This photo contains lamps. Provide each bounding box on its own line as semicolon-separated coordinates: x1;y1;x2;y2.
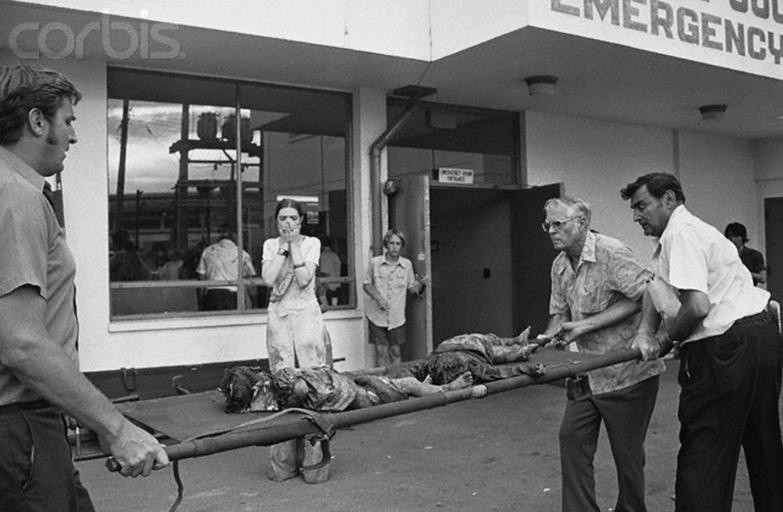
699;104;727;122
521;74;559;98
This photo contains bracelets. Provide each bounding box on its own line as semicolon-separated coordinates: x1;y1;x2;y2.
278;248;289;258
666;334;686;348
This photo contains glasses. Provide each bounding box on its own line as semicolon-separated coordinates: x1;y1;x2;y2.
542;215;581;233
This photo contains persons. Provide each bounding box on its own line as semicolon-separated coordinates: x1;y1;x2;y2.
0;62;170;511
262;201;334;482
319;236;342;304
361;229;428;369
198;232;255;310
222;325;535;417
724;221;765;286
108;225;152;283
537;197;663;509
621;172;780;511
157;249;183;279
